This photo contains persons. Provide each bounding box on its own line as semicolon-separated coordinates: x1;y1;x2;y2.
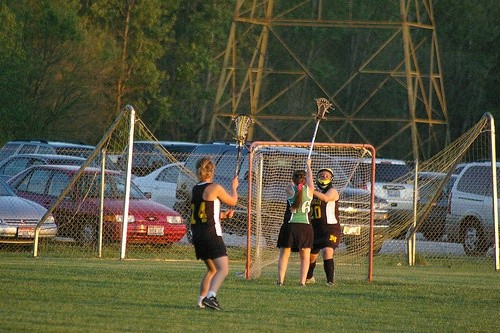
305;166;341;286
189;157;239;311
276;158;315;287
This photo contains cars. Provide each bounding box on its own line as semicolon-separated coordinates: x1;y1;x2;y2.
0;178;58;247
411;171;459;239
133;162;188;210
0;154;102;183
5;164;188;249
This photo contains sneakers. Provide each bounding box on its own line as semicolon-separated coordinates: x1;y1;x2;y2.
202;297;221;310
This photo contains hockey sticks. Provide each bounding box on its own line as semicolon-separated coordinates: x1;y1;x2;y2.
226;113;256;221
303;97;336;173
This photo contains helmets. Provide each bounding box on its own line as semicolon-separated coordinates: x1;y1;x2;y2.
316;168;334;188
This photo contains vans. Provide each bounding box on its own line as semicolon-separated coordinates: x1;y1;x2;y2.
443;162;500;256
114;139;211;176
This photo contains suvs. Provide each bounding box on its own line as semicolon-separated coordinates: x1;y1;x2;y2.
333;156;420;238
173;140;390;256
0;136;120;171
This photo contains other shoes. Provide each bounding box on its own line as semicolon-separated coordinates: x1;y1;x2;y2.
325;282;333;286
306;278;315;283
